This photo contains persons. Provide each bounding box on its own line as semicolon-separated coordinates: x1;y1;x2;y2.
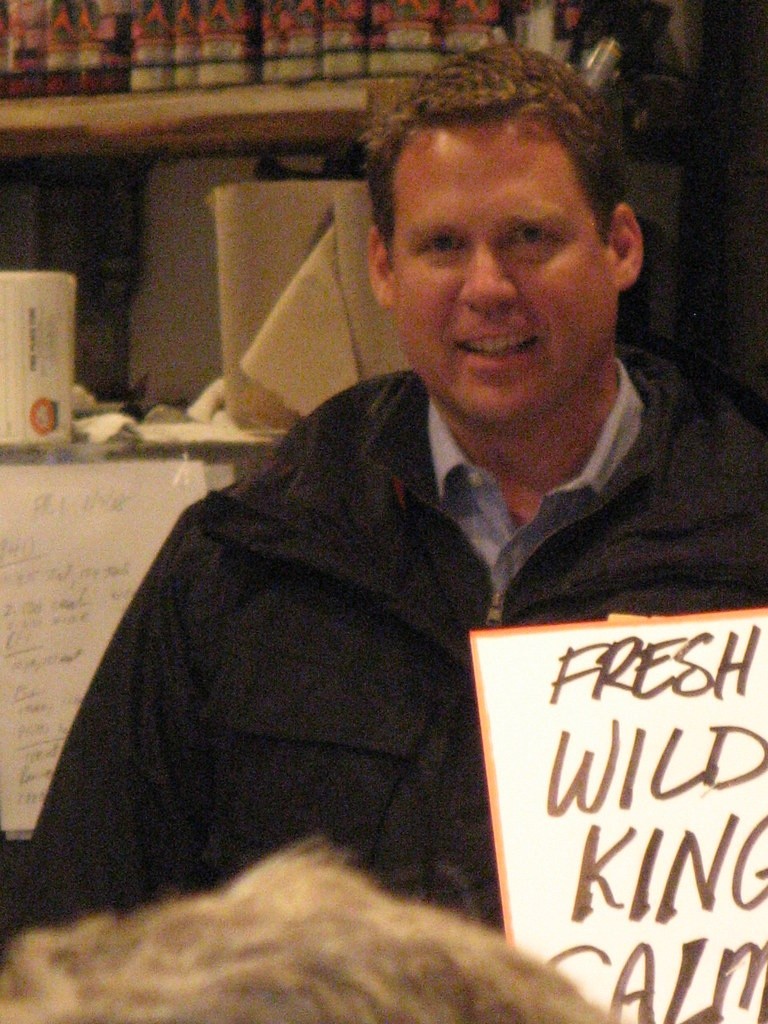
0;835;617;1024
0;43;768;929
